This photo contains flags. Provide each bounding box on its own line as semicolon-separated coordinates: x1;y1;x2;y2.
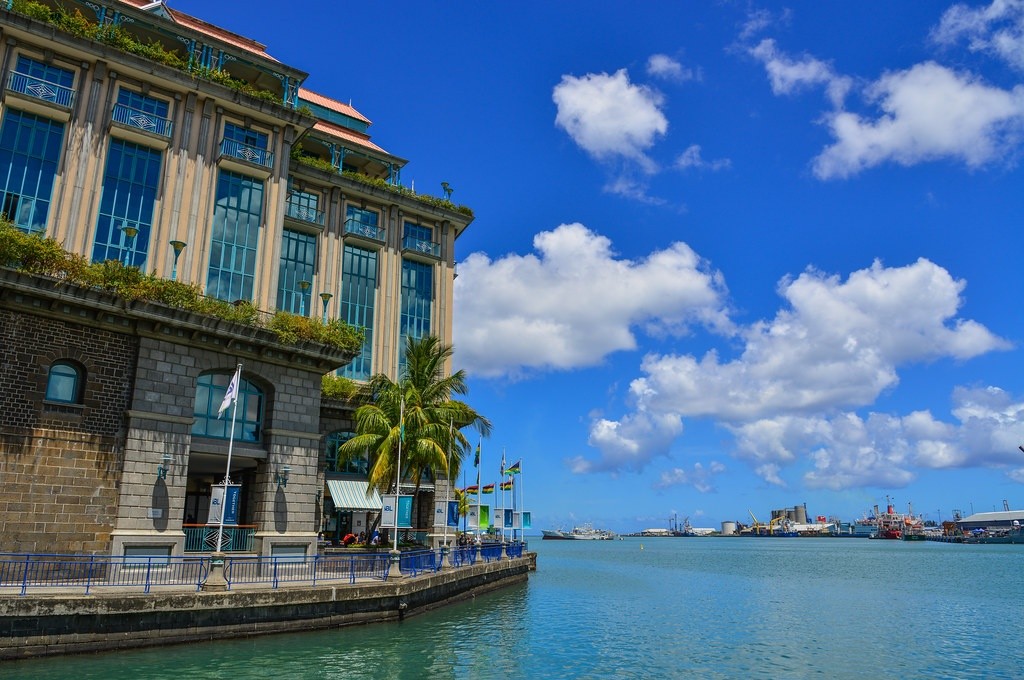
500;479;514;491
505;460;520;481
217;370;238;420
466;485;478;495
477;470;479;484
482;483;495;494
474;442;480;467
500;454;506;475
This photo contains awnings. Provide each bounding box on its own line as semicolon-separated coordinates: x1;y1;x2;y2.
326;479;383;513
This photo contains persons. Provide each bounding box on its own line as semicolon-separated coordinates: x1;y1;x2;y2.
458;532;477;562
371;526;379;544
322;518;330;538
345;533;358;546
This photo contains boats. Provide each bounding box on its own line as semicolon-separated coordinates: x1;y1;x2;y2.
671;524;698;537
540;520;624;541
867;495;927;542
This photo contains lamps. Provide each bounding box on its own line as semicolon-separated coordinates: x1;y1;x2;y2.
274;467;292;493
158;454;177;483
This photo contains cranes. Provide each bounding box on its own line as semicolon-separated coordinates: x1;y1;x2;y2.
770;515;786;537
748;510;760;535
